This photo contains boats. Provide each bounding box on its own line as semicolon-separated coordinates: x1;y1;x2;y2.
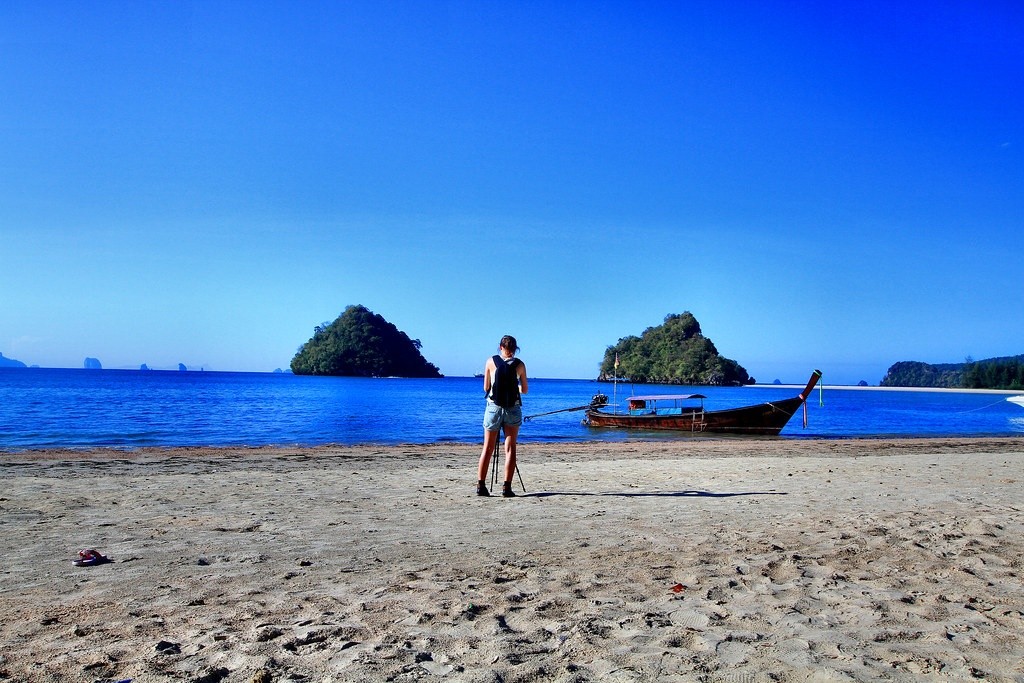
587;369;823;436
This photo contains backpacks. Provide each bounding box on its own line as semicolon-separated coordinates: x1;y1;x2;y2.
490;355;522;410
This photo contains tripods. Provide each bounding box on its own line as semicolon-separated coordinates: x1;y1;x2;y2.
490;430;526;492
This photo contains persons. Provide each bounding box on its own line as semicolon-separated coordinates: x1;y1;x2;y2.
476;334;528;497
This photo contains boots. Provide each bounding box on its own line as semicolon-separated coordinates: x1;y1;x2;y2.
502;481;516;497
477;480;490;496
